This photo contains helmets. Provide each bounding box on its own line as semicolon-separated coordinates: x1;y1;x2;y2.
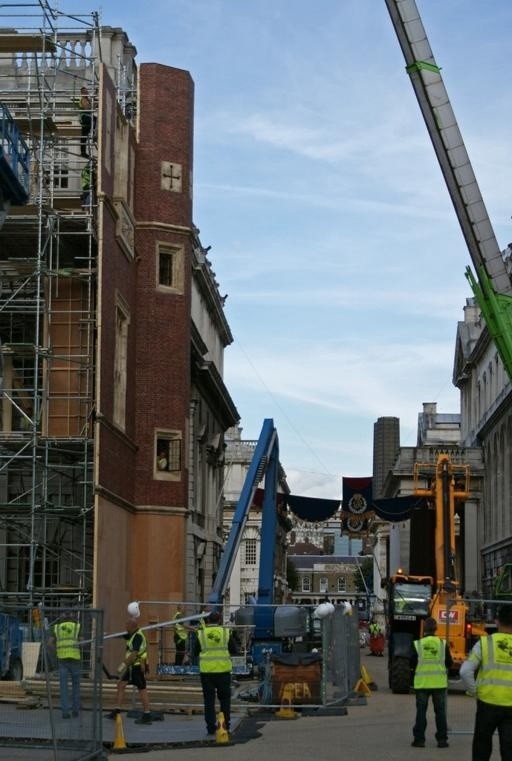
315;602;335;617
343;602;352;615
127;602;140;617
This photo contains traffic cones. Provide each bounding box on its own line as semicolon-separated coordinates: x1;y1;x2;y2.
275;684;299;719
110;714;128;751
215;713;232;745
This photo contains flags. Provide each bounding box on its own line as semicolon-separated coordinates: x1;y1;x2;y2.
341;477;375;521
339;512;370;539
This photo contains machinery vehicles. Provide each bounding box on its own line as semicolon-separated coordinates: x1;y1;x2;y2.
156;415;323;683
382;453;512;697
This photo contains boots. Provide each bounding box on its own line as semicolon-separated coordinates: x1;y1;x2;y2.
104;708;120;720
135;712;153;725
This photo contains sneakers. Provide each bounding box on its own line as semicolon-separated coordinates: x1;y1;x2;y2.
63;712;79;718
437;743;449;748
411;742;425;747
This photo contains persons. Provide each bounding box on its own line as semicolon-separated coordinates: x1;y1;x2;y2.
408;617;455;749
189;603;219;665
48;616;85;720
191;611;237;743
79;158;98;211
102;618;152;725
172;604;195;664
157;451;168;471
459;603;512;760
16;406;35;430
368;619;381;638
78;87;97;157
